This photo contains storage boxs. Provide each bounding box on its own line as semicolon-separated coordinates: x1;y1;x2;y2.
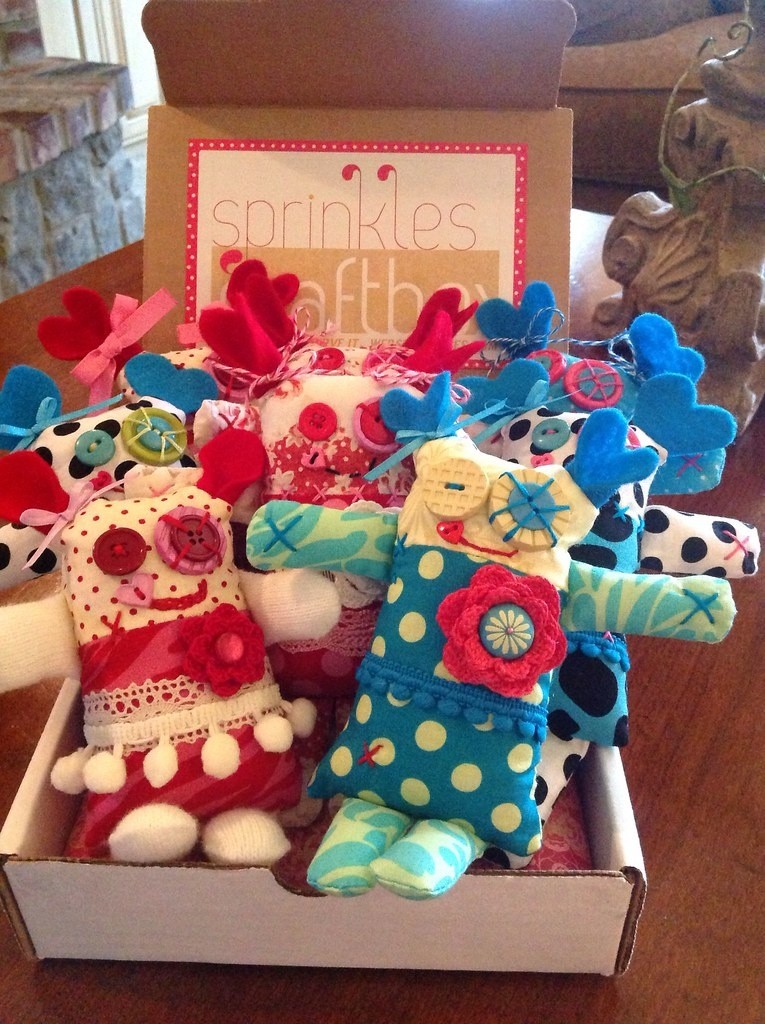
0;0;650;979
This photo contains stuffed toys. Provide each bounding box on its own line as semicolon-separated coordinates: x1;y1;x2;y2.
3;262;765;898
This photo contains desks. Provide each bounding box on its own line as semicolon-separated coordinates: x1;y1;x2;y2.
0;206;765;1024
